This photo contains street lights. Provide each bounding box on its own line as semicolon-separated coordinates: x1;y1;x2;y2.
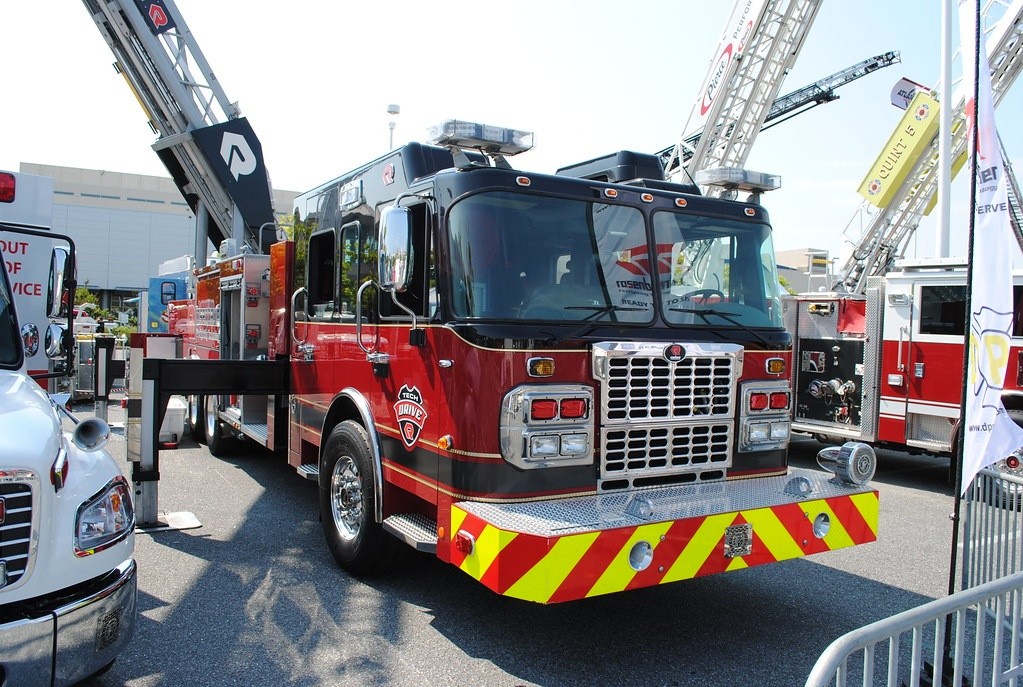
387;104;400;151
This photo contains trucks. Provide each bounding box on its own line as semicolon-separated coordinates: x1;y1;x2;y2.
0;170;138;687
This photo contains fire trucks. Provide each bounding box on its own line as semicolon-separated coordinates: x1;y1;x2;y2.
85;2;902;605
692;0;1023;512
